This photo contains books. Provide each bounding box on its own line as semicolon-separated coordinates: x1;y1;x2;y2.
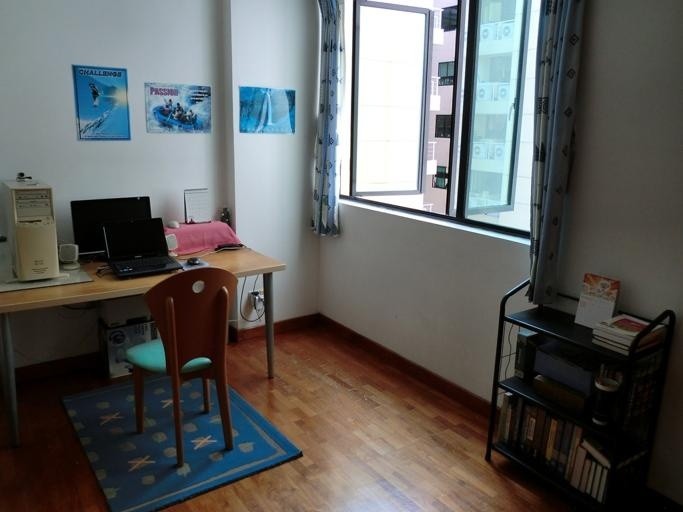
574;273;667;356
496;392;645;502
595;363;653;418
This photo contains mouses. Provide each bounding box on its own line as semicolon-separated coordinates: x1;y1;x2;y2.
188;257;200;265
167;220;179;228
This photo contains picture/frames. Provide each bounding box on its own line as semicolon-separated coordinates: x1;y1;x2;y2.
72;64;131;140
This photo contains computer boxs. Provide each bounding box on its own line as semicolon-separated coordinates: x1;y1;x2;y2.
1;178;60;282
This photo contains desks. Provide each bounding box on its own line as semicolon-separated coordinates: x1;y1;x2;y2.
0;244;288;448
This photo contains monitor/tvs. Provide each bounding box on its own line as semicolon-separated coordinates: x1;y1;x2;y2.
69;195;152;262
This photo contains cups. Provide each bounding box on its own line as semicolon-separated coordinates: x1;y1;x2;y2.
591;375;619;426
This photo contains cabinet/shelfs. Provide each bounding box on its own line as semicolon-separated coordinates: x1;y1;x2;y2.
485;277;676;512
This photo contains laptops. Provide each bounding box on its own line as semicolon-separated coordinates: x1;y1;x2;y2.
101;217;183;278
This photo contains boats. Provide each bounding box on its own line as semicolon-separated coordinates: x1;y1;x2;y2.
153;106;204;132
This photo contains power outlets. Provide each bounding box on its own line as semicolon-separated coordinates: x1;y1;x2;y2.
248;290;264;308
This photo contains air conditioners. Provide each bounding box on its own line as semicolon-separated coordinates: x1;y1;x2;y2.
472;22;515;218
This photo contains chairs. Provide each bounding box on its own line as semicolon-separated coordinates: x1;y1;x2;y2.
126;267;239;467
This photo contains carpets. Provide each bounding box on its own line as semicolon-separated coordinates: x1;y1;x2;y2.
60;375;303;512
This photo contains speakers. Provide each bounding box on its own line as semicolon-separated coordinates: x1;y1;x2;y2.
165;233;179;257
58;244;80;270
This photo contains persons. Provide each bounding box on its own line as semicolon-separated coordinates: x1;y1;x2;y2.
161;96;197;123
89;82;99;100
